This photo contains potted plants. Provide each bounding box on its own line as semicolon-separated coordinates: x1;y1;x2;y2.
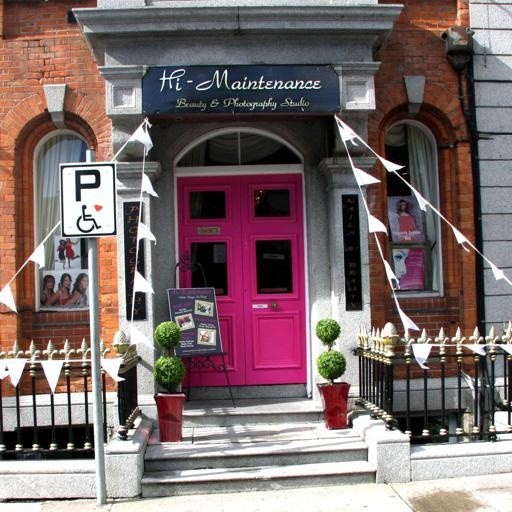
154;320;187;441
315;317;351;429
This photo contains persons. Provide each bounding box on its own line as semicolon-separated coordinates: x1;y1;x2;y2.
394;199;419;243
42;274;58;306
199;331;212;343
63;237;81;268
62;273;90;307
44;272;72;306
393;248;411;290
54;239;67;269
